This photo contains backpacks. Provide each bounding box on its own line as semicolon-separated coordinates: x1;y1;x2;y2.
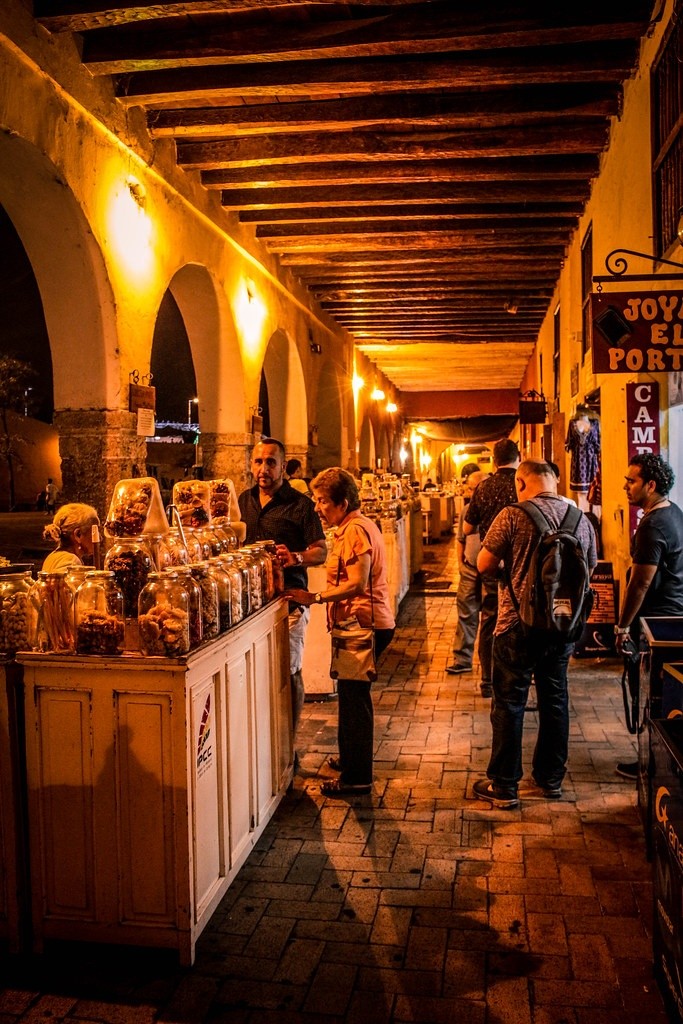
502;499;595;644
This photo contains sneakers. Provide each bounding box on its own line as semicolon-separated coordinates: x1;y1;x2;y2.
531;769;561;798
472;777;518;809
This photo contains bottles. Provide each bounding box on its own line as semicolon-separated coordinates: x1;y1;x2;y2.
0;571;76;655
68;526;285;655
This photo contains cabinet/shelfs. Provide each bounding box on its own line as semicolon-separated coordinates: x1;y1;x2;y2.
14;596;296;967
301;493;465;700
636;617;683;1024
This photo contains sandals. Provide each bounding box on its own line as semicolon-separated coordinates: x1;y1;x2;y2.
320;779;372;794
328;756;345;771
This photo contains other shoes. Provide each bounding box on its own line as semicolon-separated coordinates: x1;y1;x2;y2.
616;763;640;779
446;665;473;674
480;682;492;698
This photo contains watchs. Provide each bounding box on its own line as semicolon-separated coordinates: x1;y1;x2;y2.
614;625;631;635
292;552;305;567
314;592;324;604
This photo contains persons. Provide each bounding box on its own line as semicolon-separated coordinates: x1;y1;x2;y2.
280;467;396;797
42;503;103;573
423;479;437;492
615;453;683;780
285;458;314;499
238;439;328;771
36;478;61;517
445;438;600;808
563;402;602;493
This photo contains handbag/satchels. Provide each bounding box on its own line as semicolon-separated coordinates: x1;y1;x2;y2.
329;626;379;683
